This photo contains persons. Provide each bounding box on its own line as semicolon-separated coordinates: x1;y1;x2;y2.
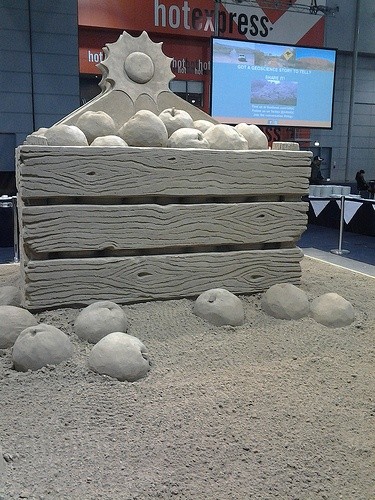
311;155;327;185
356;170;374;200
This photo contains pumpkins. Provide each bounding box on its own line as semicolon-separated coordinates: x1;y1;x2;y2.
0;304;37;349
0;285;20;304
88;330;151;380
194;288;245;327
32;106;267;149
74;299;128;343
311;294;353;326
262;282;313;319
11;323;73;371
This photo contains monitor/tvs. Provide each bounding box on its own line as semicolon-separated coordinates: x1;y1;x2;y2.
209;36;338;130
